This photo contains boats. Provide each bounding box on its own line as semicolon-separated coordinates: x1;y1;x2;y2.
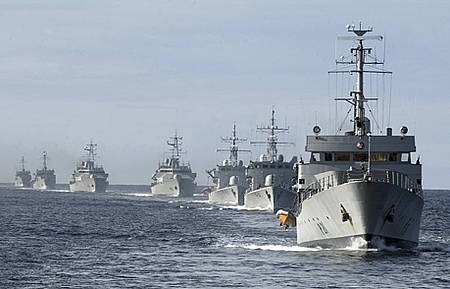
13;156;32;187
242;110;298;213
276;210;296;228
290;22;428;256
68;140;108;194
205;121;246;207
31;151;56;189
149;129;196;197
